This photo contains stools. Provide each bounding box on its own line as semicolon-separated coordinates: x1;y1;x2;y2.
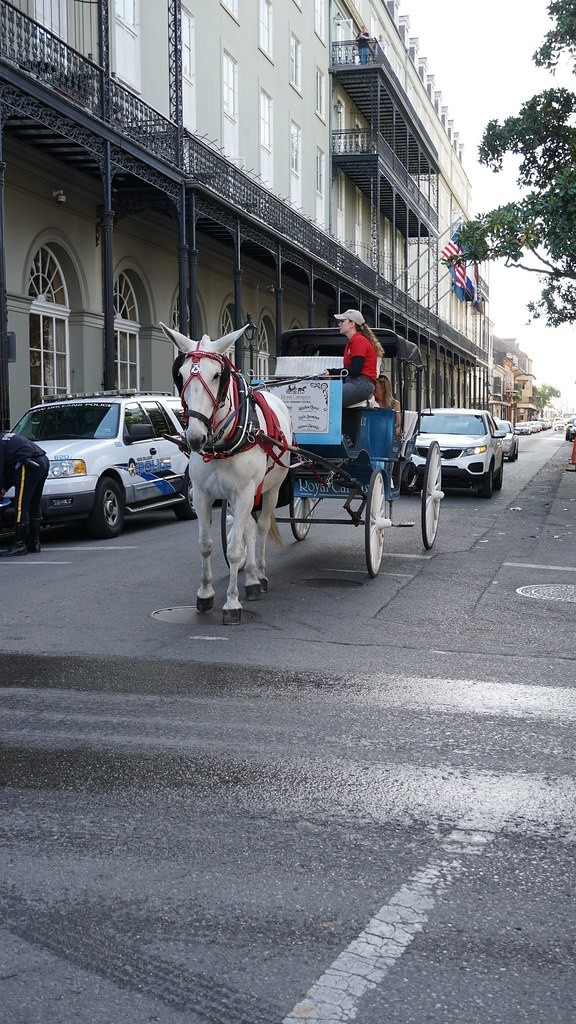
18;60;56;81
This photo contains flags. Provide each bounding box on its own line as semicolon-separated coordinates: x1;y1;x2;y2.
441;224;475;301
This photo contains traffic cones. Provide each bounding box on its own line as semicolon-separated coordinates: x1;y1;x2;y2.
566;438;576;472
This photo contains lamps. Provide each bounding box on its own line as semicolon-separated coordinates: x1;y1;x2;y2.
334;100;343;113
333;12;343;26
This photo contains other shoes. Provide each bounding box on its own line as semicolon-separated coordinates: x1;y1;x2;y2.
343;432;353;447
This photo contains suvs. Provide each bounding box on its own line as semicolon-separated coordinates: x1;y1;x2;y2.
0;388;198;539
404;407;506;498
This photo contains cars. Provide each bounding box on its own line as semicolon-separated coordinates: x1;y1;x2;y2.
497;419;519;463
513;415;576;443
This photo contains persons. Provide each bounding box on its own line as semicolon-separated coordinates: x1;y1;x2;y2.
373;375;401;435
0;433;49;557
322;309;383;446
356;26;370;65
375;34;386;62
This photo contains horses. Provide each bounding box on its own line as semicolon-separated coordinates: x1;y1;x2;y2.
157;321;293;626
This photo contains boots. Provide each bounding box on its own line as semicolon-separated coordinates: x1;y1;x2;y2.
26;519;40;553
0;525;27;556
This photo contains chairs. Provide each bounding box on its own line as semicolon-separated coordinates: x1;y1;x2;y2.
42;415;76;440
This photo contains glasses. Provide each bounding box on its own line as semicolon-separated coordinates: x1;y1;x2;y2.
379;375;388;378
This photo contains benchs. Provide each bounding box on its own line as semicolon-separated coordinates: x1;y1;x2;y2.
398;409;418;459
274;356;380;409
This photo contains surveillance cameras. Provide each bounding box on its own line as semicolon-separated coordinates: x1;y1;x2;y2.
270;288;274;292
58;194;66;203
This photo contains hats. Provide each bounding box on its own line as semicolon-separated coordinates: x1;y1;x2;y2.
334;309;365;326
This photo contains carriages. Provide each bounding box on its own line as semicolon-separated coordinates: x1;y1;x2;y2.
158;320;444;627
